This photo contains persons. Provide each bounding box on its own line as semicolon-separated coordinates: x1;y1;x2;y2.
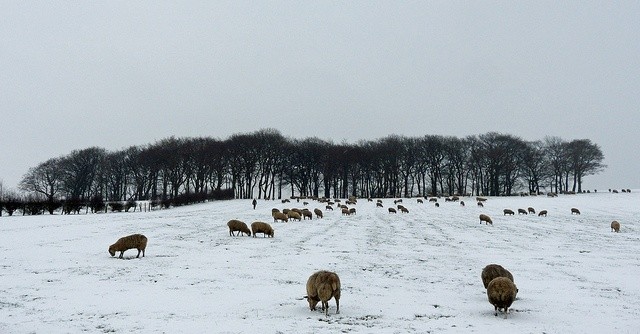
252;198;258;210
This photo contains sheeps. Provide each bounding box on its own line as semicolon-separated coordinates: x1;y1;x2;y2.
487;277;519;319
611;220;620;233
528;207;535;214
314;208;323;218
283;208;291;214
303;270;341;316
273;212;288;223
518;209;527;215
480;214;492;225
272;208;280;216
287;211;301;221
282;196;334;210
571;208;580;215
504;209;514;216
481;264;513;289
409;188;631;207
538;210;547;217
108;234;148;258
251;222;274;238
334;194;409;216
292;208;302;216
227;220;251;236
302;210;312;220
